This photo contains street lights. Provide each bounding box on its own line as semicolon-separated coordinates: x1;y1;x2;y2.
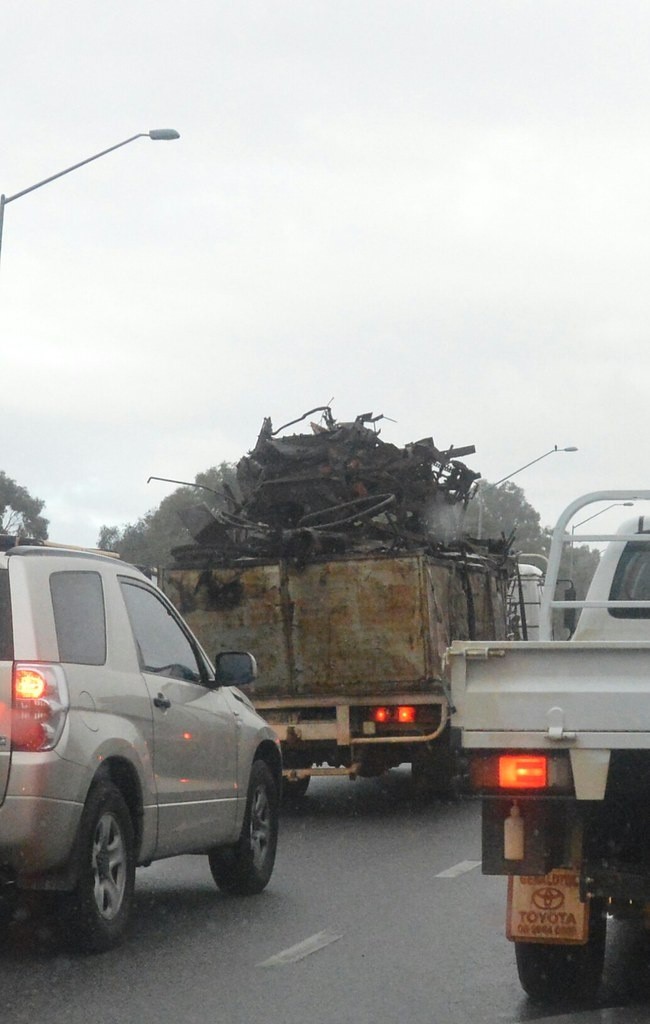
476;445;578;541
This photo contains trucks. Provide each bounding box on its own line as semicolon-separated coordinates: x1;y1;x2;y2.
155;551;577;802
446;490;650;998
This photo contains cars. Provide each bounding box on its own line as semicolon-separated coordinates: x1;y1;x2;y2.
1;535;283;952
